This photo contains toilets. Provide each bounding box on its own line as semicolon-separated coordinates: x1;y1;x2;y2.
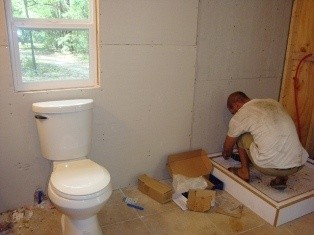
31;99;113;235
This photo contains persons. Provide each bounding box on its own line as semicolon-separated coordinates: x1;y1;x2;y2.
222;91;309;190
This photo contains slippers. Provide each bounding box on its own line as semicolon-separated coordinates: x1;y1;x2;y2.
270;179;287;188
228;167;250;182
